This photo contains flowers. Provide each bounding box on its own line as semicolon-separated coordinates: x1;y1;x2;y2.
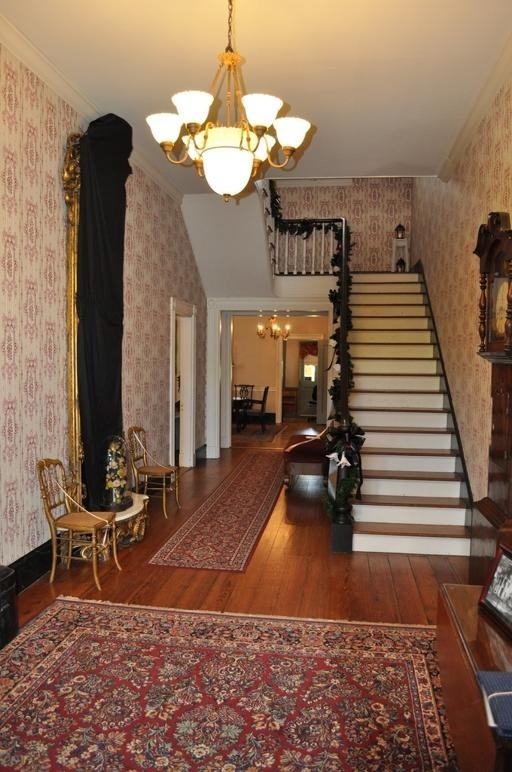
103;440;128;489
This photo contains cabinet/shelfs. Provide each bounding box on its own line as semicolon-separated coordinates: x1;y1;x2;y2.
0;565;20;649
390;237;410;274
431;583;511;772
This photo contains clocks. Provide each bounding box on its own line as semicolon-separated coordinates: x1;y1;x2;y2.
465;208;511;582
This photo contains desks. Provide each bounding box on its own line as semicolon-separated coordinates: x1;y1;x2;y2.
106;488;151;549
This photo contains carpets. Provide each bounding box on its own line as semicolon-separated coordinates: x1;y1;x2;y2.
0;594;458;772
231;423;290;445
144;447;286;573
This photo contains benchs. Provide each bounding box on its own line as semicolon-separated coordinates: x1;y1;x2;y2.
282;421;334;491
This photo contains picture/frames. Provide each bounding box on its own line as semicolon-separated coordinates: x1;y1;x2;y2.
477;544;511;644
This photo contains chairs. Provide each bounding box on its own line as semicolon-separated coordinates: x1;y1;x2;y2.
282;386;300;418
127;425;182;521
37;458;124;593
231;382;275;434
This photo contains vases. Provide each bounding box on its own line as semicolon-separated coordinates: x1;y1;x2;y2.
111;487;123;504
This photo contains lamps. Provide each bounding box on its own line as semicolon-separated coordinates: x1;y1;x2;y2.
145;1;312;202
257;317;291;343
395;223;406;239
